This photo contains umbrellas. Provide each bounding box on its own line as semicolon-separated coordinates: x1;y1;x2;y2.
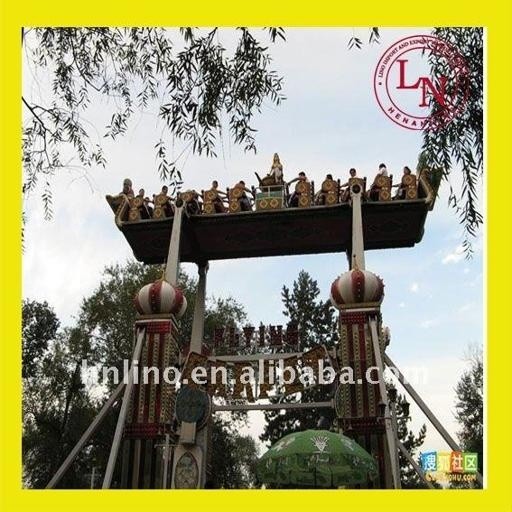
254;428;381;488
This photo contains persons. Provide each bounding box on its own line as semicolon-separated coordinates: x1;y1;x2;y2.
135;162;412;221
267;153;285;184
